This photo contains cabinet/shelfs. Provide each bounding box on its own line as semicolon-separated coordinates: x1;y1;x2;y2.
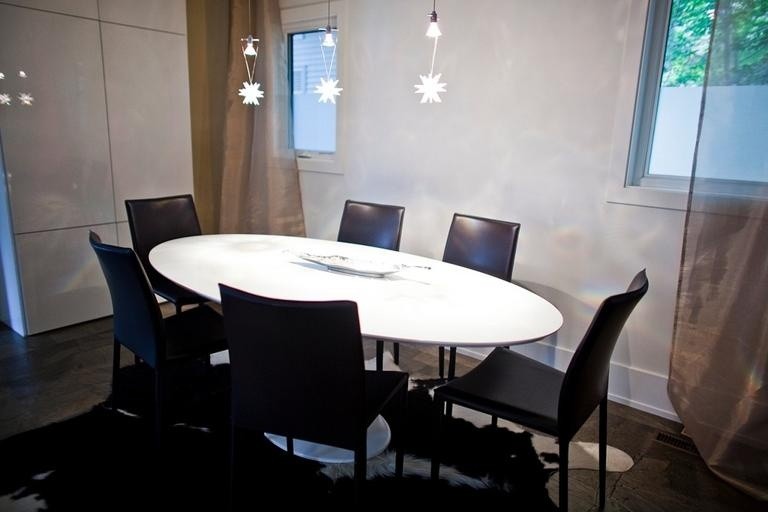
0;0;196;235
0;221;170;340
0;1;187;36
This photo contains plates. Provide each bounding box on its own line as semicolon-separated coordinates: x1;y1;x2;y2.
299;252;401;278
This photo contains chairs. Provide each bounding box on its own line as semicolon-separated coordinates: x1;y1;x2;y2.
124;194;212;316
87;228;227;445
336;199;406;374
428;268;649;511
437;211;522;381
217;282;411;512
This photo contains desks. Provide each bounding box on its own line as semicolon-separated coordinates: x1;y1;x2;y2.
145;232;563;486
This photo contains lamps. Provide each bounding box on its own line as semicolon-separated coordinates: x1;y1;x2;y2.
312;0;345;106
411;1;448;105
237;1;265;107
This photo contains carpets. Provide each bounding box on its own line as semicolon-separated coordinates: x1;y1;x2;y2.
0;349;636;511
